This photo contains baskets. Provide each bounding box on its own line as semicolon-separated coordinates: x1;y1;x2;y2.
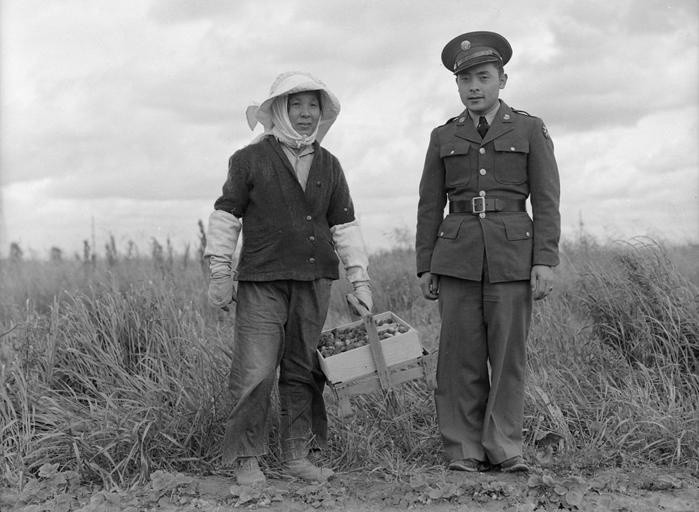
314;291;425;400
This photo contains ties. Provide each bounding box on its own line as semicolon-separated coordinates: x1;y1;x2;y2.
476;116;491;137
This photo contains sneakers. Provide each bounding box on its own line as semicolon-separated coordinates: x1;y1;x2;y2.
280;457;337;483
499;454;530;473
448;455;491;474
233;455;267;486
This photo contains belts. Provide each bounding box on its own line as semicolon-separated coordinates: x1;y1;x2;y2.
446;197;527;215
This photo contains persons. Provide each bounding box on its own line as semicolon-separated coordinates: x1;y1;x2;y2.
413;30;562;475
204;68;373;486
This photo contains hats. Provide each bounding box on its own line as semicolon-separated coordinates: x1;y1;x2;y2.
245;68;342;142
441;30;514;76
